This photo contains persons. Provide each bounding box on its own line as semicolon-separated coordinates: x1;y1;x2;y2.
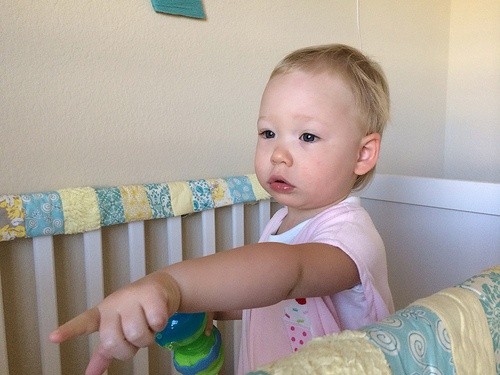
46;43;395;375
